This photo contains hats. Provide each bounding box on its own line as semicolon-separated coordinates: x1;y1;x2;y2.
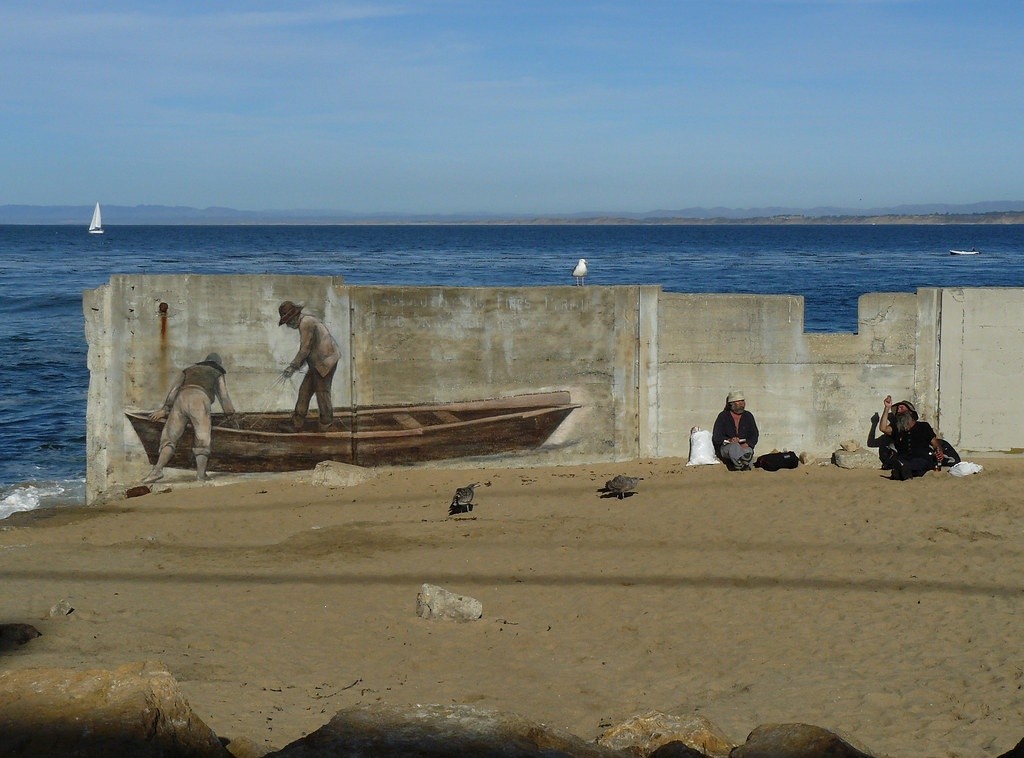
728;389;746;402
891;400;919;421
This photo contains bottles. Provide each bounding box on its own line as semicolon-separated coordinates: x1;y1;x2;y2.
933;446;943;472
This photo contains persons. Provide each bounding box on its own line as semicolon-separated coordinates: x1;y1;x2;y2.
713;388;759;472
879;395;944;481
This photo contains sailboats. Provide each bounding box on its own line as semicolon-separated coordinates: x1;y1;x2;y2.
87;200;104;234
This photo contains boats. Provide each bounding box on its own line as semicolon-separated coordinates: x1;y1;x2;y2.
949;250;979;255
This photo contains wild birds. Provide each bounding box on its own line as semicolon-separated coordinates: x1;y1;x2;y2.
570;258;589;287
452;482;481;513
605;475;644;500
